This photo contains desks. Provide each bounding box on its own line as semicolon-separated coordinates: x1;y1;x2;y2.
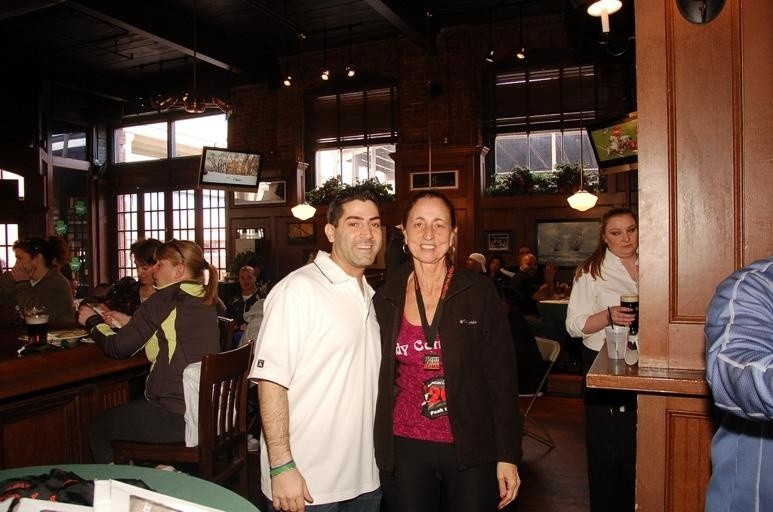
538;297;569;334
0;463;262;512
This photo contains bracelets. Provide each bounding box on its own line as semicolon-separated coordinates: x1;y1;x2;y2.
266;459;297;480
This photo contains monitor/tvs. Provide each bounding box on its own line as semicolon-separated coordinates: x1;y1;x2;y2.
198;146;262;193
586;111;637;168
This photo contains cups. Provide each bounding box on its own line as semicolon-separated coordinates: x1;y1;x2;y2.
607;358;626;377
24;306;50;347
604;326;629;360
619;293;639;336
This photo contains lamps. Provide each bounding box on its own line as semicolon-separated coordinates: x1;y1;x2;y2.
319;29;333;81
342;24;358;80
565;1;598;213
482;0;496;63
133;3;235;119
514;0;528;61
289;26;316;221
586;0;623;33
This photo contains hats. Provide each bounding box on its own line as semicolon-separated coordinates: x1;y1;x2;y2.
469;252;487;273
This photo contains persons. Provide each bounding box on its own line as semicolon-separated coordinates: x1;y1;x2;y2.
701;257;773;512
0;234;164;404
78;239;224;465
564;208;638;512
209;262;306;454
246;191;388;512
466;245;576;400
370;188;524;512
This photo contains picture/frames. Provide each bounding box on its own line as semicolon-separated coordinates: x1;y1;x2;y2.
229;177;288;208
483;230;513;255
533;218;603;270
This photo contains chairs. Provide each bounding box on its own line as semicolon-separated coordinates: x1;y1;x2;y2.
216;314;235;353
518;336;561;449
499;284;523;317
110;340;255;498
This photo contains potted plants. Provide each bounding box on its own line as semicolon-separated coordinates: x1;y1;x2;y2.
486;161;606;197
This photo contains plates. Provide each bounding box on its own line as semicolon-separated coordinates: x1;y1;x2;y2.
17;329;89;346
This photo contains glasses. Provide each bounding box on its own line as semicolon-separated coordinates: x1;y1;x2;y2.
16;238;39;254
166;238;185;264
